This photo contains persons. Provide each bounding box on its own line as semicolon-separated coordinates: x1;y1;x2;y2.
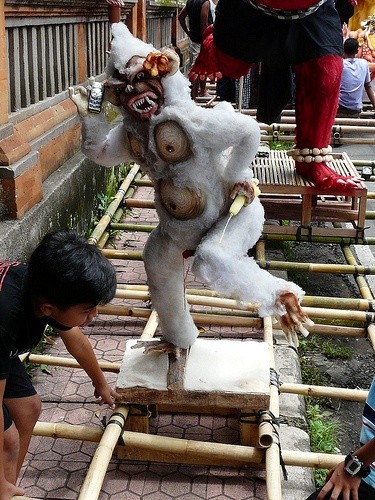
306;376;375;500
178;0;218;101
0;227;125;500
336;38;375;119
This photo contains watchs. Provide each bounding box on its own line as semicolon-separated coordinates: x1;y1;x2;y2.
344;452;371;478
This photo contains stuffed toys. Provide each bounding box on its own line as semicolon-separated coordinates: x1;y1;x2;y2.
69;21;313;354
190;0;367;191
